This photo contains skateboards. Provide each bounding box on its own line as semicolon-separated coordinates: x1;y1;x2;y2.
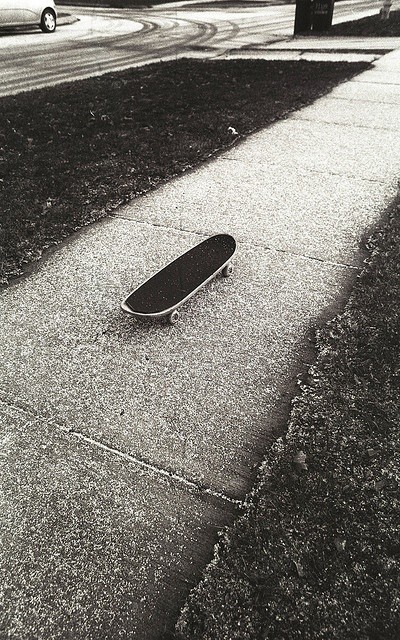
120;233;238;325
